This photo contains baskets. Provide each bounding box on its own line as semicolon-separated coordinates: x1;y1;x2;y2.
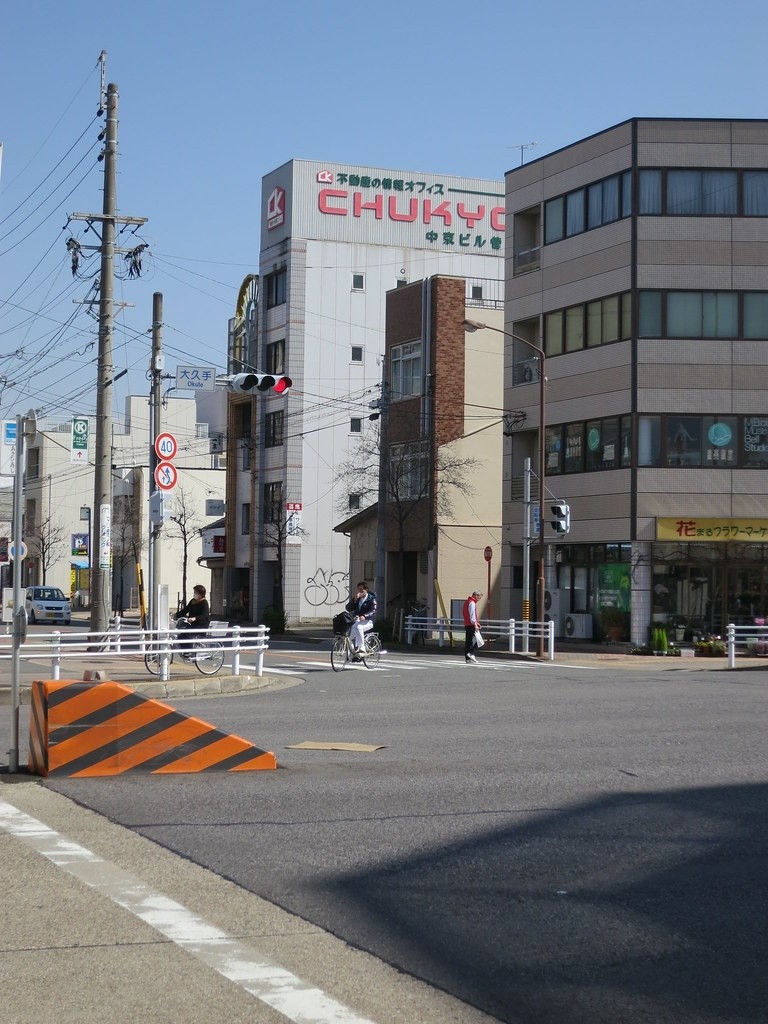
333;622;351;637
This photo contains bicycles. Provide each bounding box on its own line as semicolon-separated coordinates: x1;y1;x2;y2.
331;612;383;671
143;613;225;676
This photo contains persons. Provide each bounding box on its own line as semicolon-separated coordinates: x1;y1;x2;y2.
345;581;378;663
462;590;484;664
172;585;210;658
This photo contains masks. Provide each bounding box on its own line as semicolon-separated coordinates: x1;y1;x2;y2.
193;594;200;599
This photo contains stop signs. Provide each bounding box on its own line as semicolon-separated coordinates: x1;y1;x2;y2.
484;545;492;561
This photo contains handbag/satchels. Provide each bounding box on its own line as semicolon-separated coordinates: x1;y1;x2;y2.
474;629;485;648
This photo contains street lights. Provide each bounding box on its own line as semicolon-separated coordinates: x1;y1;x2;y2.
460;319;547;661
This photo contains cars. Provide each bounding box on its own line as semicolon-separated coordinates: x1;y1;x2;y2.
24;586;72;625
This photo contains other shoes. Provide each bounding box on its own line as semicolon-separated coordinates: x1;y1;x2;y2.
466;659;478;664
183;652;191;658
357;648;366;653
467;653;477;662
332;611;356;633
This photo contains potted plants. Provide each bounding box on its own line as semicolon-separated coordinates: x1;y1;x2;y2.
601;607;625;642
651;628;727;657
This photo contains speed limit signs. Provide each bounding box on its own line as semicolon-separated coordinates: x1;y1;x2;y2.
155;432;178;462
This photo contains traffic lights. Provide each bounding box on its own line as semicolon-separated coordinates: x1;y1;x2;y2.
232;373;293;396
551;504;571;536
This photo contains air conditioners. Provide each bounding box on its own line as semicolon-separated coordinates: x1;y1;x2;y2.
543;587;571;638
565;612;592;639
